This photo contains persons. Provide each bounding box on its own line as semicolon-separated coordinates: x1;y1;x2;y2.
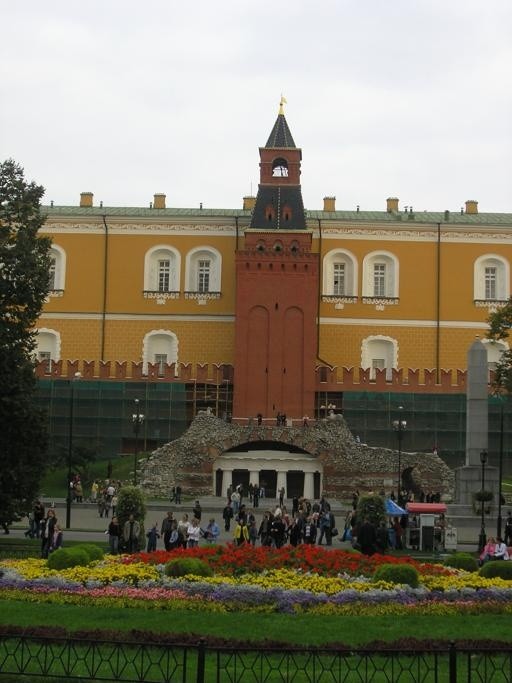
193;501;202;523
70;474;83;503
23;496;63;558
222;483;336;548
504;511;512;547
90;479;123;519
340;487;446;557
0;514;10;534
105;514;220;556
493;537;509;561
478;536;496;566
170;485;182;505
217;410;309;427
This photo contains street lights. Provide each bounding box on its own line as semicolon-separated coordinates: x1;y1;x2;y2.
477;447;490;555
128;395;147;488
318;402;337;415
392;404;409;503
65;370;86;529
